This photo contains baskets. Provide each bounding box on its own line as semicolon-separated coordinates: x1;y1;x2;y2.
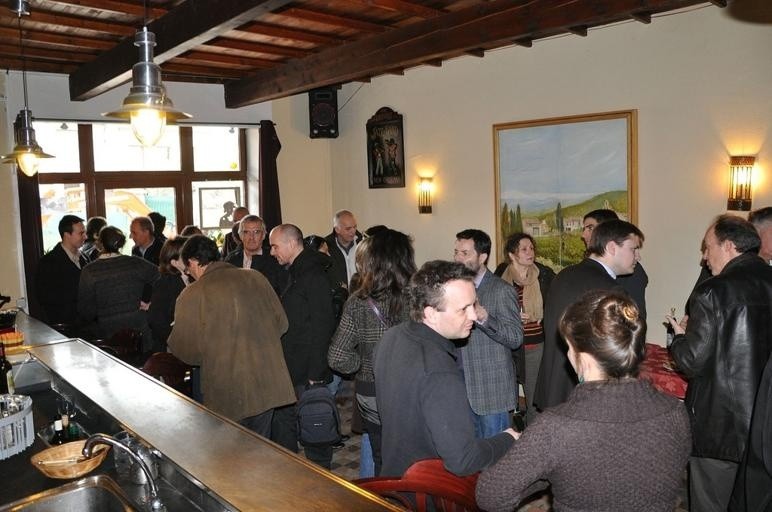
31;439;111;479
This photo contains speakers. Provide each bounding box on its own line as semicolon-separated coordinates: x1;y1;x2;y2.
308;87;339;139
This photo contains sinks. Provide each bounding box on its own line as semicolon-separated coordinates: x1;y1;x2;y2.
1;474;142;512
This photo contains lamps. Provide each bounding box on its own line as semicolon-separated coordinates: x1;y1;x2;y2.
101;0;193;150
1;0;57;179
417;177;433;215
727;156;755;211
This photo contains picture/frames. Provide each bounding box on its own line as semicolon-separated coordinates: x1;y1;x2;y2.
366;107;406;188
492;109;638;275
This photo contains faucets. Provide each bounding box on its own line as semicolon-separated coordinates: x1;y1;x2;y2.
82;432;168;512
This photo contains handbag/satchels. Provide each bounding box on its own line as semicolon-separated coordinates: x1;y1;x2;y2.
295;384;341;447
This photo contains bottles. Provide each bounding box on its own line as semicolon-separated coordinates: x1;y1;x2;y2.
53;415;67;445
127;440;159;484
1;342;16;396
666;307;678;349
65;415;79;442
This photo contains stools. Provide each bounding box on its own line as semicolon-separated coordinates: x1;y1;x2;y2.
49;321;93;343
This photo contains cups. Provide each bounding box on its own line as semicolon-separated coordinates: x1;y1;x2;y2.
113;432;136;461
56;394;77;417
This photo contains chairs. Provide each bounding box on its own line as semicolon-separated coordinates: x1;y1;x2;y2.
93;328;144;368
351;457;487;511
139;352;192;398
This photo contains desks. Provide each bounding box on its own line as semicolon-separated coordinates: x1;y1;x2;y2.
639;343;689;400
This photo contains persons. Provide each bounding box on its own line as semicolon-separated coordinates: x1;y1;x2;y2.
37;208;772;512
